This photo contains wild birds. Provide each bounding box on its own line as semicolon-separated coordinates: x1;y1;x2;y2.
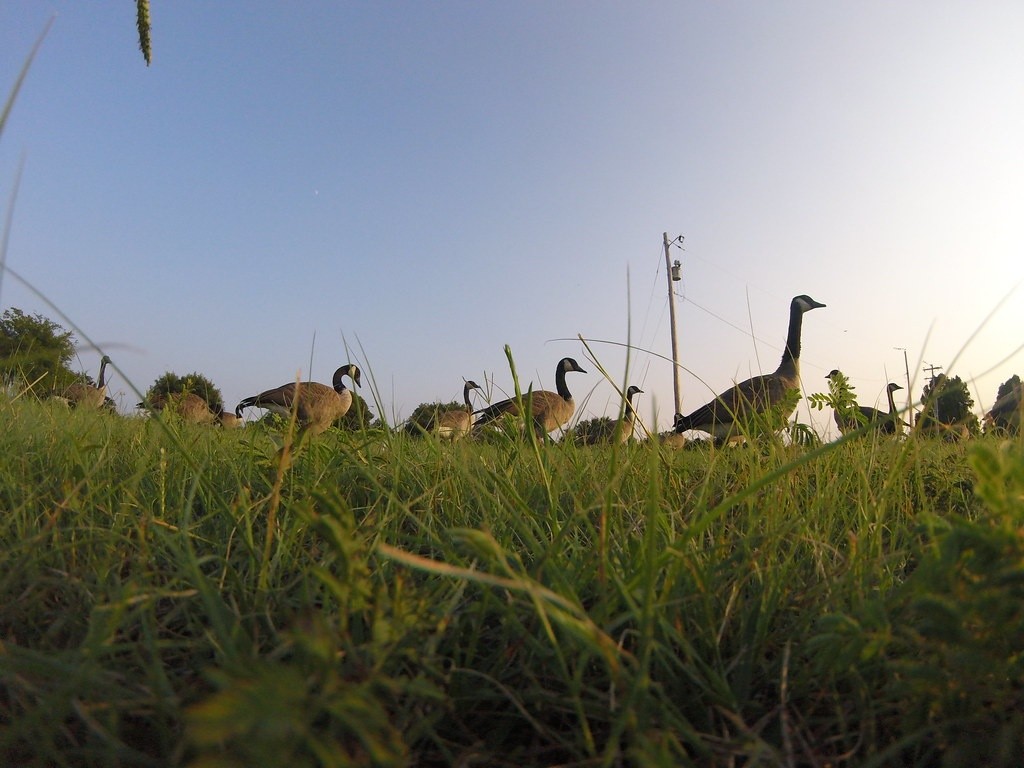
0;355;243;432
235;364;361;435
673;295;827;448
398;381;481;443
470;358;587;438
824;370;1024;442
559;386;644;448
